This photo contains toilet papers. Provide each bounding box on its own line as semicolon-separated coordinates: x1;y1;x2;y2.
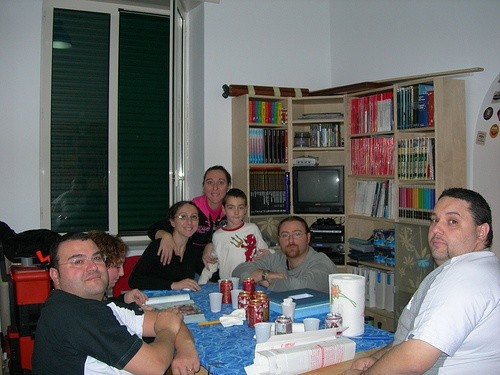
328;273;366;338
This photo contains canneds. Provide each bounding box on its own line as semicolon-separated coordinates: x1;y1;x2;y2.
220;279;233;304
275;315;293;335
242;277;255;292
325;312;344;336
238;290;269;329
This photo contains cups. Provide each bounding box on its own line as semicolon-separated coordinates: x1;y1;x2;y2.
281;302;296;323
227;277;240;289
303;318;320;332
253;322;271;343
209;292;223;314
230;289;244;310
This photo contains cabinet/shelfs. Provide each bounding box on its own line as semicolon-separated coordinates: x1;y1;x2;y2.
345;76;467;334
8;265;50;375
230;94;347;274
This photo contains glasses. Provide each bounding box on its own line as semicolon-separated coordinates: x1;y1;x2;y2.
57;253;107;267
173;215;200;221
279;231;307;239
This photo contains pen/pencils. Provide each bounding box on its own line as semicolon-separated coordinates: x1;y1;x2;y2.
198;321;220;325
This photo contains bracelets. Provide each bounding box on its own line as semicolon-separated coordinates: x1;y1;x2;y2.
262;270;271;280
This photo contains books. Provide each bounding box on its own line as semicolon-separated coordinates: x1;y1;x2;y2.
398;137;435;181
310;124;339;148
354;181;392;219
250;171;290;216
249;101;282;124
351;137;393;176
249;128;288;164
397;84;434;130
351;92;394;135
398;187;435;225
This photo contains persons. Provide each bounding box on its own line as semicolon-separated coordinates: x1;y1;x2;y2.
33;230;200;375
197;189;275;285
347;188;500;375
233;216;337;295
128;201;205;291
146;166;231;271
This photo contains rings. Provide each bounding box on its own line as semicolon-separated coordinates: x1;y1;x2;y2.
187;368;194;371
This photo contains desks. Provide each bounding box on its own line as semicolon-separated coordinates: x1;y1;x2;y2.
141;281;395;375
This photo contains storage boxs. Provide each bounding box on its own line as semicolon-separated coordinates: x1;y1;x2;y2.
267;288;330;319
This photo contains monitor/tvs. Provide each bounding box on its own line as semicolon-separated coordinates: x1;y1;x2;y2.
292;165;344;215
